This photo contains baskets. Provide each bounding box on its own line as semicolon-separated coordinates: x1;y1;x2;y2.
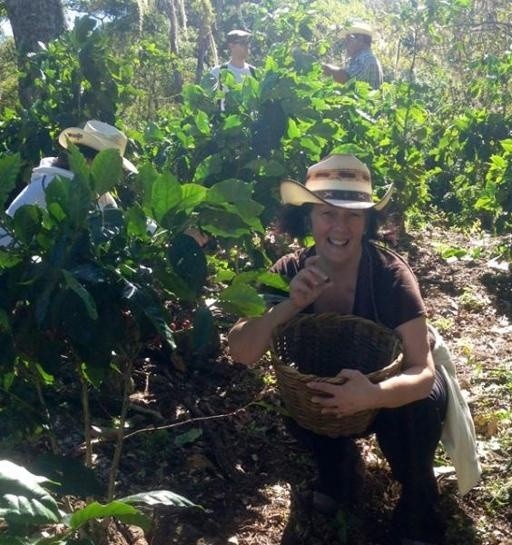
270;312;405;440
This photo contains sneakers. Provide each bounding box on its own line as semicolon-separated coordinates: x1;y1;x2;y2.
312;448;363;513
396;489;439;545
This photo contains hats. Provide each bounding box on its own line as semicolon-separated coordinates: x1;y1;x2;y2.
279;153;395;212
335;22;380;42
58;117;140;175
226;28;250;40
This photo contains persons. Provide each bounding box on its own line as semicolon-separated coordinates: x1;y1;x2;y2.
319;20;383;94
208;29;260;112
0;118;209;277
229;152;447;539
